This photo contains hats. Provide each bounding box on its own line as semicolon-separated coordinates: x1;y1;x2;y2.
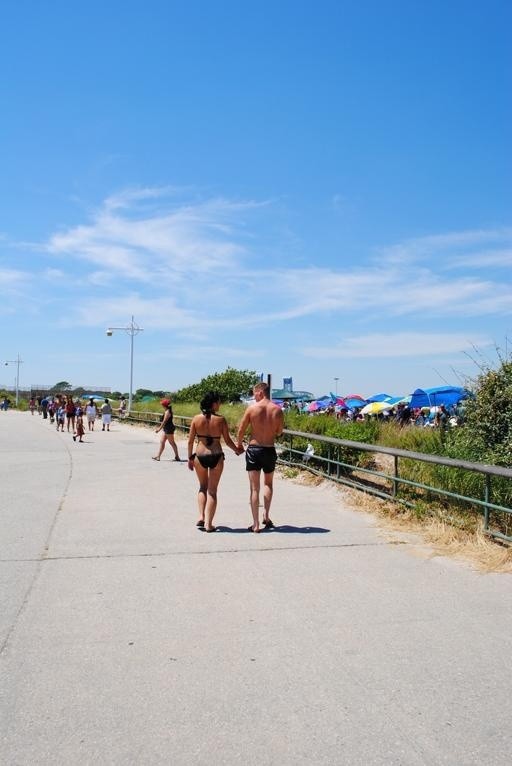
160;400;170;407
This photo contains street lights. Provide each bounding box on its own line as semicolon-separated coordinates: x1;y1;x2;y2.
3;353;24;408
106;314;144;417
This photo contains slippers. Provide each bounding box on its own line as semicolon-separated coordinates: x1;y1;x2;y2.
248;527;259;533
207;526;216;532
264;521;273;528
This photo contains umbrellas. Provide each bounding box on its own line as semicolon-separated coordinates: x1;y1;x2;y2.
271;388;406;421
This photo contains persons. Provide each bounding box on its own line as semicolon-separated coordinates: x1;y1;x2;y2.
4;396;8;411
402;404;449;429
152;399;180;462
236;382;286;534
186;390;244;533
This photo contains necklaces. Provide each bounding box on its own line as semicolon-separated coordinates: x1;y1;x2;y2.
29;392;127;443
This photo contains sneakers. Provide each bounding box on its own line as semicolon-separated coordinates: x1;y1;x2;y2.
196;521;204;529
